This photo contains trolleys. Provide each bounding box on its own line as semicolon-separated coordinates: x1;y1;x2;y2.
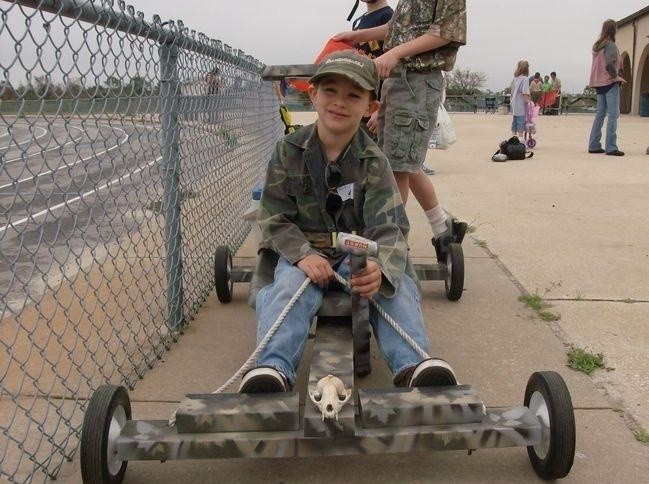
525;98;536;147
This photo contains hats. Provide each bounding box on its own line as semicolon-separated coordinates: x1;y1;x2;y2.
308;50;379;98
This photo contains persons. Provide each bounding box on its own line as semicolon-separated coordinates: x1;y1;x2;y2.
236;48;460;395
351;0;399;145
527;69;562;116
511;59;532;147
587;18;628;156
331;0;470;265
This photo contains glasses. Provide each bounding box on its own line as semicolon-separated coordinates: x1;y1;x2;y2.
325;161;342;215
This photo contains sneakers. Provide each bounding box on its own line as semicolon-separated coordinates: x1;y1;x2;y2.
408;358;457;386
431;217;467;263
238;366;286;393
607;150;624;156
589;148;606;153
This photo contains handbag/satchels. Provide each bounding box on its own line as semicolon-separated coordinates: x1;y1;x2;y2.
501;136;526;160
